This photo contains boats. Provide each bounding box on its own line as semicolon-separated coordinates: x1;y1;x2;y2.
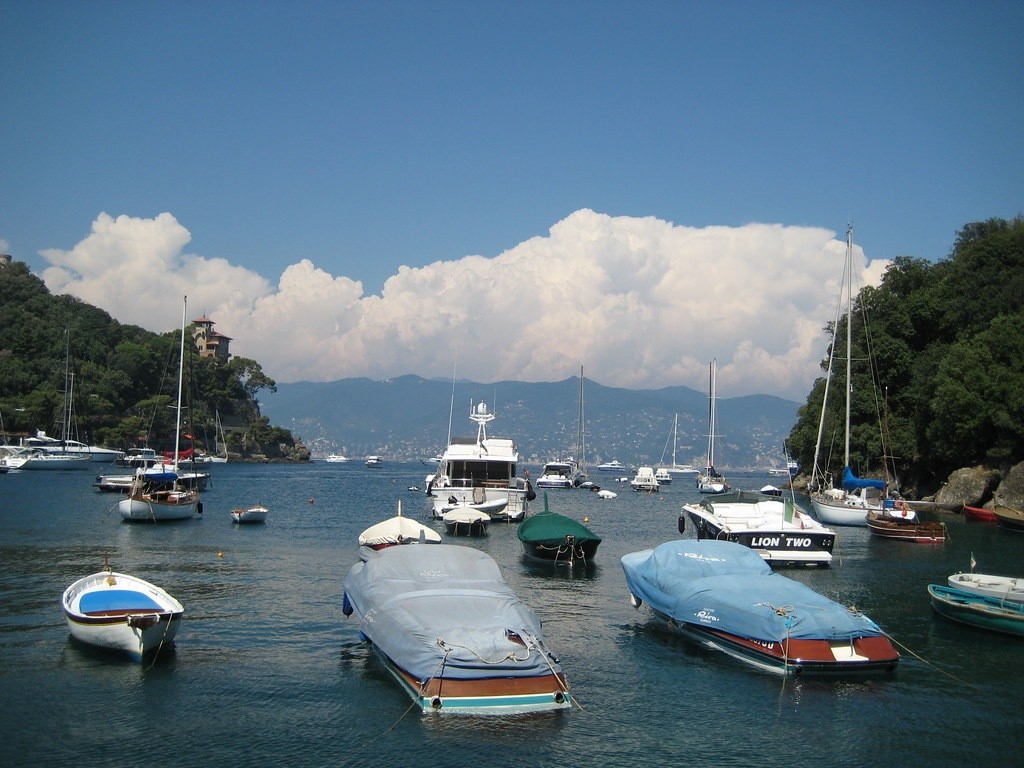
962;498;1024;533
343;543;572;715
230;502;268;522
597;459;672;491
61;552;185;666
620;484;900;679
518;490;602;563
114;445;158;468
420;363;533;535
367;456;383;467
326;455;346;463
24;429;124;461
926;572;1024;634
536;458;578;489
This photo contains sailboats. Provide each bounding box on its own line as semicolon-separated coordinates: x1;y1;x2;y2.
163;403;229;469
571;365;592;488
806;218;944;543
659;356;730;494
0;327;91;470
119;293;200;521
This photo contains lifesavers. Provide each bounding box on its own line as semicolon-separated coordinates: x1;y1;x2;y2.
696;483;698;488
701;484;703;488
565;481;570;486
197;501;204;514
895;500;906;509
